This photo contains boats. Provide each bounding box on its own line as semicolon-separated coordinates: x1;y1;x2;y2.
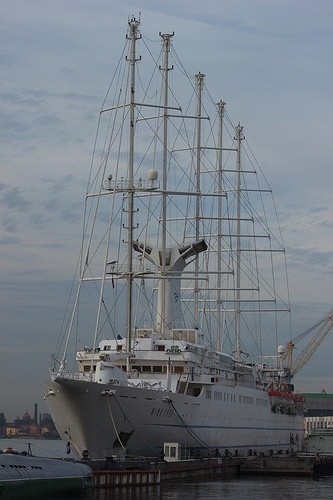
0;447;94;499
42;9;306;471
